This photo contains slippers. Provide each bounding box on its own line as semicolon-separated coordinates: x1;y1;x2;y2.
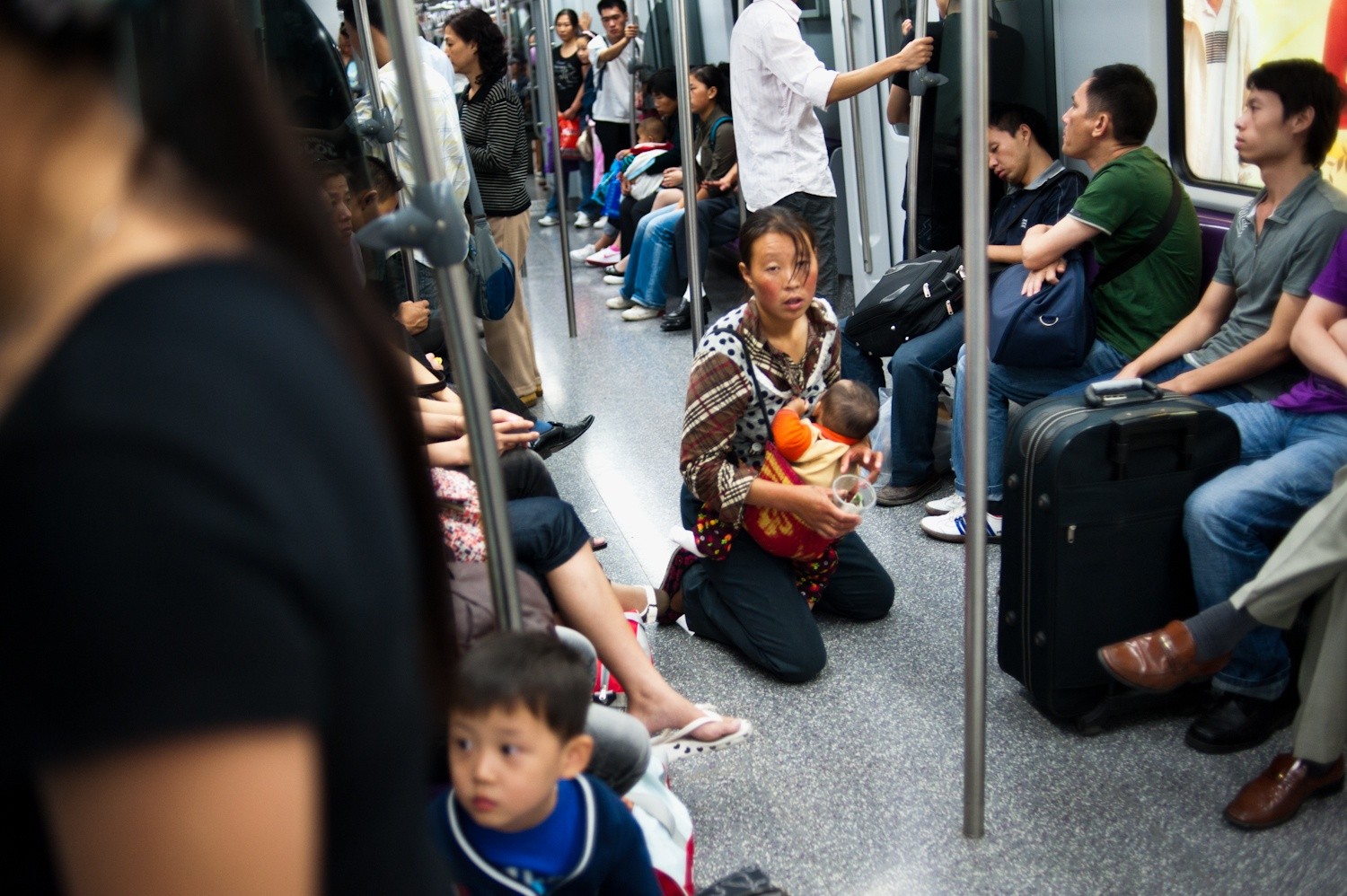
637;704;750;775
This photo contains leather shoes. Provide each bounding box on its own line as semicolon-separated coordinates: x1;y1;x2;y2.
530;415;596;460
662;293;712;320
1097;620;1233;695
1185;685;1301;756
660;312;709;331
1222;750;1346;831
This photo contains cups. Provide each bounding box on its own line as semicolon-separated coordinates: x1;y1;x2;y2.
830;474;877;530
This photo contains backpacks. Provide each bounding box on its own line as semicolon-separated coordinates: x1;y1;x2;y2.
580;65;597;111
989;251;1103;375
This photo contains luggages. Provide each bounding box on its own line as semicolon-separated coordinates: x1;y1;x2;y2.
996;377;1244;739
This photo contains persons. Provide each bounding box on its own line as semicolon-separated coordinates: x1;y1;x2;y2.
693;379;879;609
0;0;1347;896
656;205;895;680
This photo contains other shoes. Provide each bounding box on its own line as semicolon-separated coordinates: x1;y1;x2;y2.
573;211;590;228
620;304;660;320
603;264;626;277
603;275;624;285
606;296;633;309
594;215;608;228
570;244;596;262
636;583;670;640
654;546;698;625
587;533;608;552
538;216;560;226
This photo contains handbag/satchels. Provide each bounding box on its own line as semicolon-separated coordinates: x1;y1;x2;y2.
844;245;966;359
462;227;516;323
555;112;581;162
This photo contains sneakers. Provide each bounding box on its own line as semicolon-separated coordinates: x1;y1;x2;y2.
586;246;621;266
